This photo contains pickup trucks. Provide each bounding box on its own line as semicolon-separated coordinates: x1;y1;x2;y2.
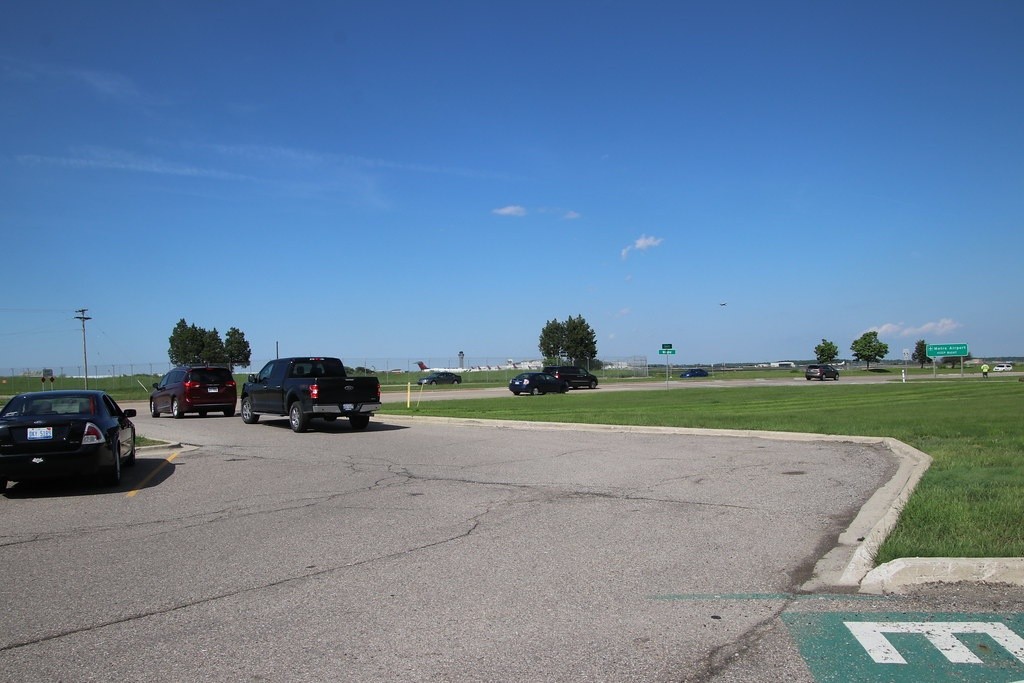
240;357;382;434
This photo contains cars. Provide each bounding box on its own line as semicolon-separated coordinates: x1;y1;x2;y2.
993;363;1013;372
418;371;462;386
508;373;570;396
680;368;708;378
0;390;137;494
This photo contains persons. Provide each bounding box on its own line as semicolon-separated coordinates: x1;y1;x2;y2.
981;362;989;378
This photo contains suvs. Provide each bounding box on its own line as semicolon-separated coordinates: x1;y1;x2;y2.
544;365;599;390
804;363;839;382
150;364;237;420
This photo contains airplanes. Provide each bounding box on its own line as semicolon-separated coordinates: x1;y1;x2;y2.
720;303;727;307
